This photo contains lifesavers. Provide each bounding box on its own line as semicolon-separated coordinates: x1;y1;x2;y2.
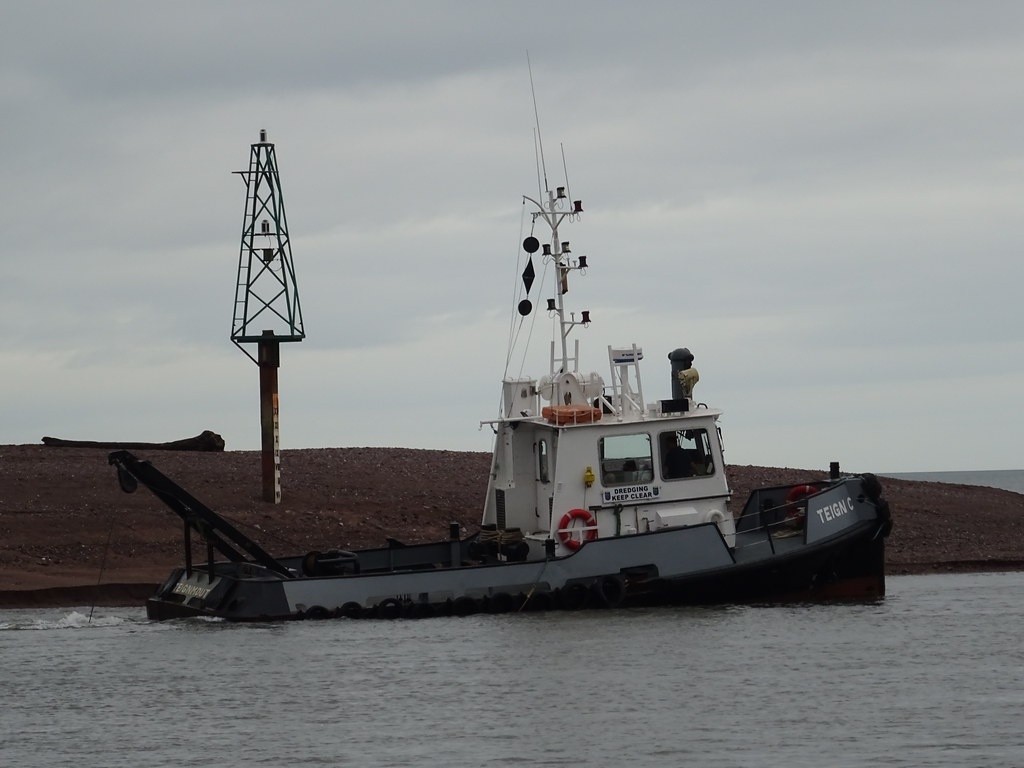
303;574;624;620
785;486;817;523
559;509;597;550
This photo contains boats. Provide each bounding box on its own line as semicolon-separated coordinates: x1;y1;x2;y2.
105;46;896;623
